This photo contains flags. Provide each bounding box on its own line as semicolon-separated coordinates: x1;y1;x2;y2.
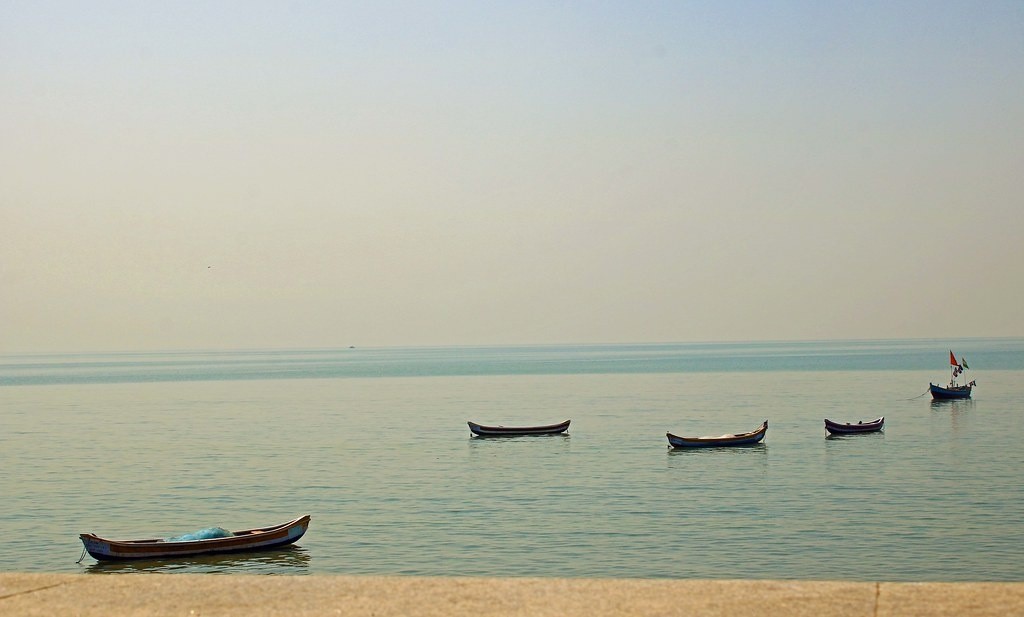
963;356;969;370
950;351;960;366
953;365;963;378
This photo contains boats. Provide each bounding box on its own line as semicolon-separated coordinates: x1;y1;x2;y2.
666;419;768;447
930;382;972;399
467;420;571;437
78;515;312;561
824;417;885;434
